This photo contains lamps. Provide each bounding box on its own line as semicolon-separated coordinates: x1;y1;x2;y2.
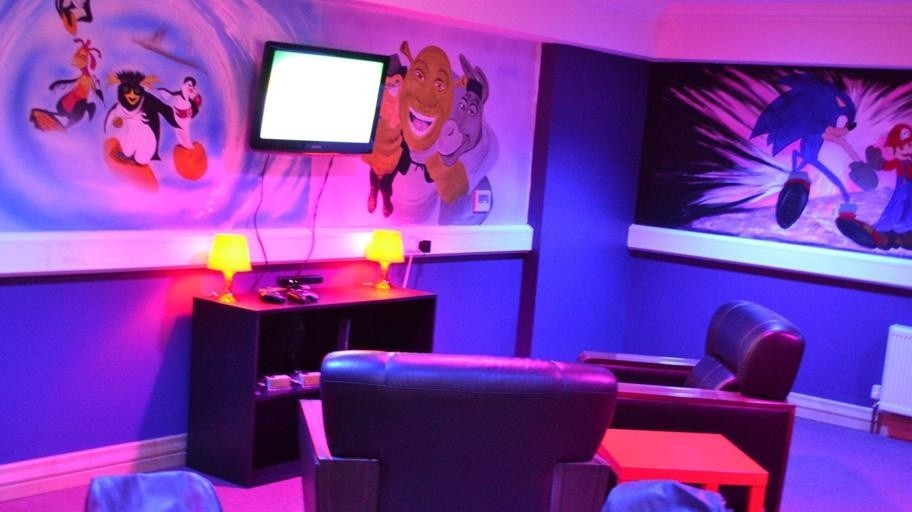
207;233;252;306
365;229;404;290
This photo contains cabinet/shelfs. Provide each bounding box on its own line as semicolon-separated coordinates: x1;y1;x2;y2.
187;283;438;488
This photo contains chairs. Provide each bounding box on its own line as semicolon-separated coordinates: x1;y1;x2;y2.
85;469;223;512
577;299;805;512
299;348;618;511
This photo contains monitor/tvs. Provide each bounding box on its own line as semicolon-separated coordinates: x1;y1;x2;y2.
249;40;391;155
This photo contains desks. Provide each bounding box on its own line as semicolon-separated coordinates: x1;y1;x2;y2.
598;427;769;512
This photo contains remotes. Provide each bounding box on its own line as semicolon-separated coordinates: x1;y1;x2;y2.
260;290;287;304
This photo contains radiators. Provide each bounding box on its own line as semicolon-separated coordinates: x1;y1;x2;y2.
879;324;911;420
